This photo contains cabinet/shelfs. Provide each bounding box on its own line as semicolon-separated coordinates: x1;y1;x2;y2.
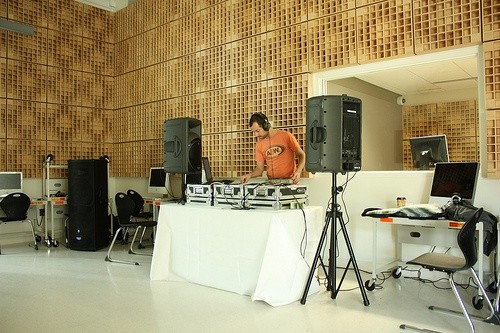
42;154;109;246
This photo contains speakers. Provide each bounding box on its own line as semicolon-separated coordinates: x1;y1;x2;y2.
163;117;202;175
66;159;110;251
305;94;362;174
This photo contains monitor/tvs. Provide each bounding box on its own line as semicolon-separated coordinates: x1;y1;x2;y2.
0;172;23;197
429;162;480;208
148;167;169;198
409;135;449;170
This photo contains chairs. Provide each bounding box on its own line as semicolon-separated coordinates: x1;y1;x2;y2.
401;207;500;333
0;192;38;255
105;188;157;266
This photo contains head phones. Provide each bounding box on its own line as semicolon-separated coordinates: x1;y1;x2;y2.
256;112;271;131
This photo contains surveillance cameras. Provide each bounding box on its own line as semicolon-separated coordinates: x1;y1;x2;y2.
396;96;407;105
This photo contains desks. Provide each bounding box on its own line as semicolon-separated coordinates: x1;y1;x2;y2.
32;201;52;248
150;202;323;308
363;215;500;310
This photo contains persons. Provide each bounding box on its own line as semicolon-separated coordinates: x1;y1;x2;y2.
240;112;305;183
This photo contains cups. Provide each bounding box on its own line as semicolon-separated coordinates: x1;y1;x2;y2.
397;197;406;208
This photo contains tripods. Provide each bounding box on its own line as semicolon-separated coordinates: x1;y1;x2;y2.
301;172;370;305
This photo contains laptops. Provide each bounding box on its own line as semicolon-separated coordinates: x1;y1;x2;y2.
202;157;233;183
